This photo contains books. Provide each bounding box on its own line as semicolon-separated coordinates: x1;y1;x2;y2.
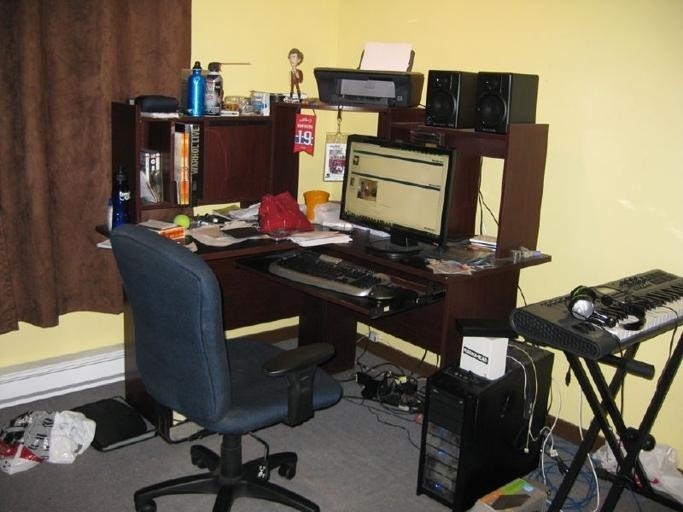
138;220;184;239
139;122;201;207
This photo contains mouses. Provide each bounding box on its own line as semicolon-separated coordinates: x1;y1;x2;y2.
370;281;403;302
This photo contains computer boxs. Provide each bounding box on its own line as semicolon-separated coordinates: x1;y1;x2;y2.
416;339;555;512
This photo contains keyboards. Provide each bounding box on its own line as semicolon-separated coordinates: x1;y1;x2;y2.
268;245;388;298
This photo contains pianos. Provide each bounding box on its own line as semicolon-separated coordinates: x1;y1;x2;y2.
513;267;683;359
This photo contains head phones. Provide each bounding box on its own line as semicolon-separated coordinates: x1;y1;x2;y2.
569;284;647;334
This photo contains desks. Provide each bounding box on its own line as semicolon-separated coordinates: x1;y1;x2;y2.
234;97;551;395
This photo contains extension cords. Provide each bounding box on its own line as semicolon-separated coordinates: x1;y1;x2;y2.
360;392;425;414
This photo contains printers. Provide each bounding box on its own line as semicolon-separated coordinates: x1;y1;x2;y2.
313;41;425;110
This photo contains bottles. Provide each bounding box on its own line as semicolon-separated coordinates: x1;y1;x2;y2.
111;163;132;230
204;61;224;117
186;61;207;118
106;198;115;233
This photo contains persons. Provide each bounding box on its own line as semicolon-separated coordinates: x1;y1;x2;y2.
285;47;305;100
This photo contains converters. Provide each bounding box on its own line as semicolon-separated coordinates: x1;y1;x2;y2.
398;377;415;395
357;371;379;393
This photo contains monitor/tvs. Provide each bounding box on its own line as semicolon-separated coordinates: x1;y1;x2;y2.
339;133;459;261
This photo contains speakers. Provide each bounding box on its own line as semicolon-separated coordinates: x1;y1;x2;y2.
425;69;478;130
473;69;539;137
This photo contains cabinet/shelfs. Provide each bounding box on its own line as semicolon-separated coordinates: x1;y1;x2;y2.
140;125;191;227
202;123;277;243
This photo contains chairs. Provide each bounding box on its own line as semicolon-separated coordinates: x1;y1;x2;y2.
109;225;342;512
